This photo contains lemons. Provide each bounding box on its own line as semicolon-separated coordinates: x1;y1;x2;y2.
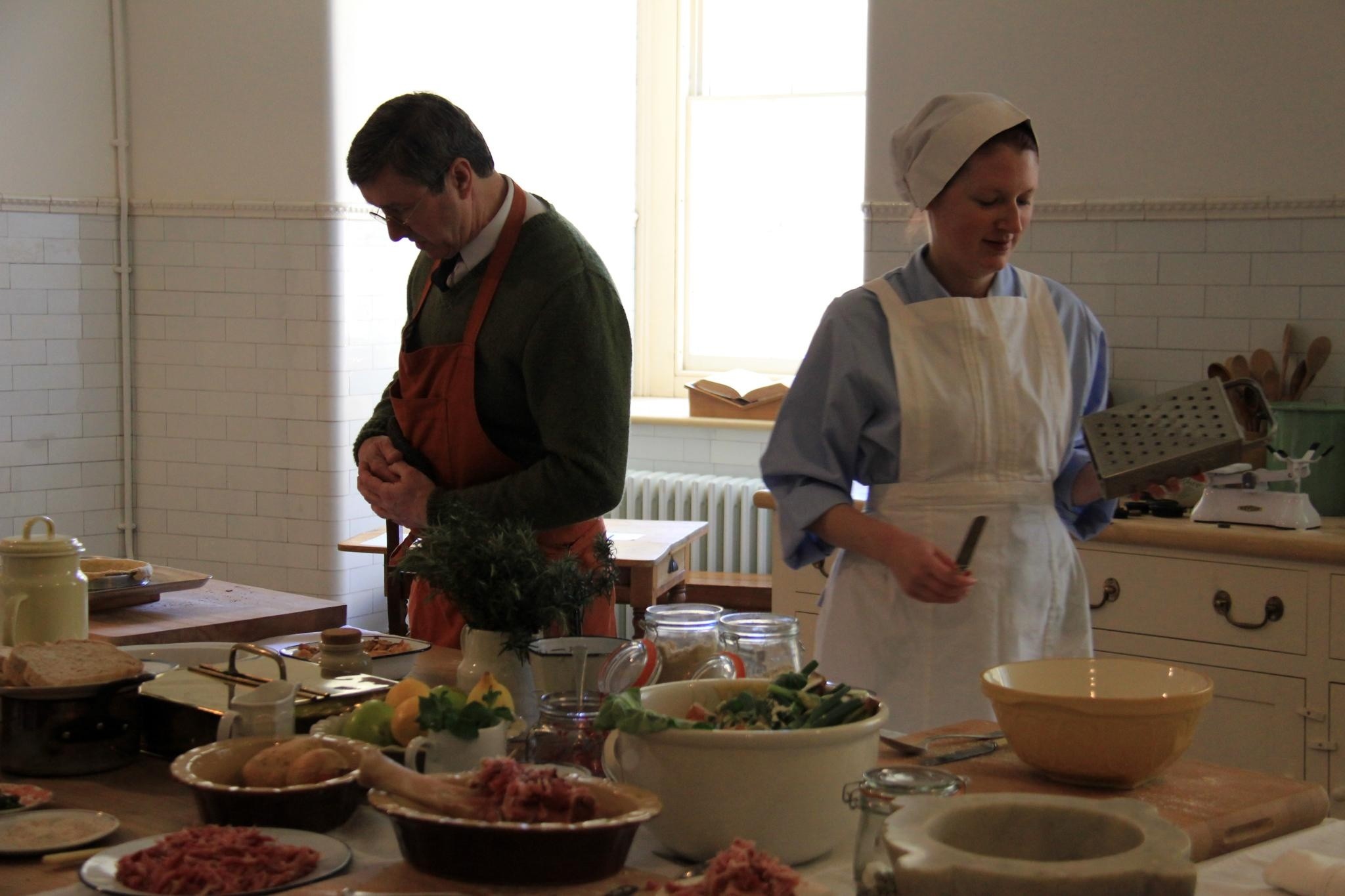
341;671;515;748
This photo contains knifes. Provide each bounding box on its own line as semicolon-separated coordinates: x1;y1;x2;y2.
952;517;992;572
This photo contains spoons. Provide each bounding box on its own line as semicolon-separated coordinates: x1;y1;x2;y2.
878;727;1004;754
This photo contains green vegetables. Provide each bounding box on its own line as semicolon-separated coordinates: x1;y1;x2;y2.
591;657;866;735
391;499;620;665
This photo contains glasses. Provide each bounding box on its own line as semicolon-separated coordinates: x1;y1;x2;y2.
368;165;451;233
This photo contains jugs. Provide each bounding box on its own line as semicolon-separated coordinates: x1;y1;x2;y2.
217;679;302;741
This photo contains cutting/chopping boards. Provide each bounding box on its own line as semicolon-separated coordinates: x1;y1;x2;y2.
877;719;1331;861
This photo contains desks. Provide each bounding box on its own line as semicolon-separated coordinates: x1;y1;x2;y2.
339;516;710;635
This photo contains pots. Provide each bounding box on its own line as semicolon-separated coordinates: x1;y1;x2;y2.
0;657;180;778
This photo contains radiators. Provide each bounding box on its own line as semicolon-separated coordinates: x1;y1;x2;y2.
607;471;769;574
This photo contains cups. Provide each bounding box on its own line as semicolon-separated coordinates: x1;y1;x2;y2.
0;515;88;647
1264;401;1345;517
404;718;506;774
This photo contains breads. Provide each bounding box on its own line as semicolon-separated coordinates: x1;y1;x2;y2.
0;640;146;687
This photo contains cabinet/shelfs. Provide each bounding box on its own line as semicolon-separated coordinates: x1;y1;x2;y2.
753;491;1344;814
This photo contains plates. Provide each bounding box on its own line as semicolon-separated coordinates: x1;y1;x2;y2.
307;712;526;757
0;810;120;853
0;783;51;810
79;830;352;896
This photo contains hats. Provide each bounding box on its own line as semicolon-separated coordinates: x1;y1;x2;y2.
887;88;1041;213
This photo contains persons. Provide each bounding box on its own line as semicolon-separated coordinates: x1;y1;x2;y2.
759;91;1209;736
347;92;632;649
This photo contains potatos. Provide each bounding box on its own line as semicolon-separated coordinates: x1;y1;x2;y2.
241;733;321;787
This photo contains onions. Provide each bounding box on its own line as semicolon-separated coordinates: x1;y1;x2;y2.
284;747;351;785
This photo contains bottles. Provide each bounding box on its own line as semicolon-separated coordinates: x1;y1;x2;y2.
843;766;971;896
308;629;373;680
526;691;611;779
641;604;801;680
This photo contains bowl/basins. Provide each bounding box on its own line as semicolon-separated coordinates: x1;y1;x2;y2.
980;657;1215;792
279;634;432;681
170;735;378;830
528;635;631;691
369;773;664;886
616;678;888;864
